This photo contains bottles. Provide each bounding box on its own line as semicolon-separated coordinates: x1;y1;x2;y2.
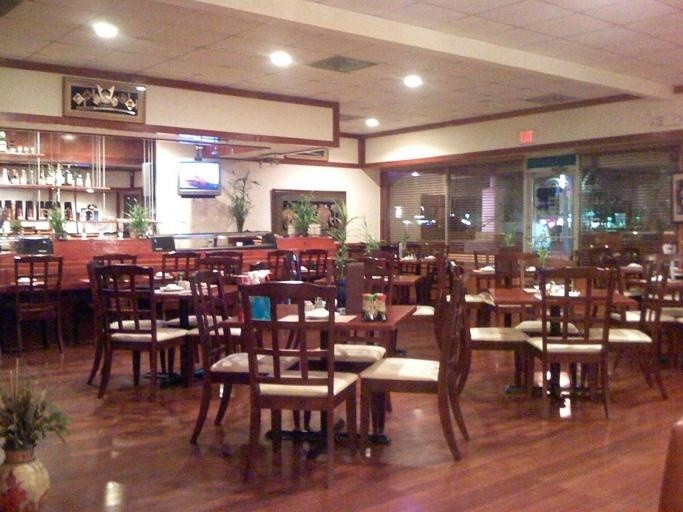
0;131;7;150
0;164;92;187
362;293;388;321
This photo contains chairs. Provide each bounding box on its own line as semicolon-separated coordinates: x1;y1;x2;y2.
12;255;66;354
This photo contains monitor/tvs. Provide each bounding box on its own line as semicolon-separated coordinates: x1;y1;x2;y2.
176;160;222;198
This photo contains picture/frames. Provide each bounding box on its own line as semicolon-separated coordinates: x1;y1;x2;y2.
671;170;683;223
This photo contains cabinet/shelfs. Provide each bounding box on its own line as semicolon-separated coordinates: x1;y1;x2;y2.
0;151;161;240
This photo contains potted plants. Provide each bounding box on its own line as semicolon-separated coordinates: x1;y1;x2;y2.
0;361;73;512
45;203;69;239
123;198;155;239
220;167;261;233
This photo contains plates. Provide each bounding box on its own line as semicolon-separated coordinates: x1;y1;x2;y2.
159;287;184;291
304;311;339;319
